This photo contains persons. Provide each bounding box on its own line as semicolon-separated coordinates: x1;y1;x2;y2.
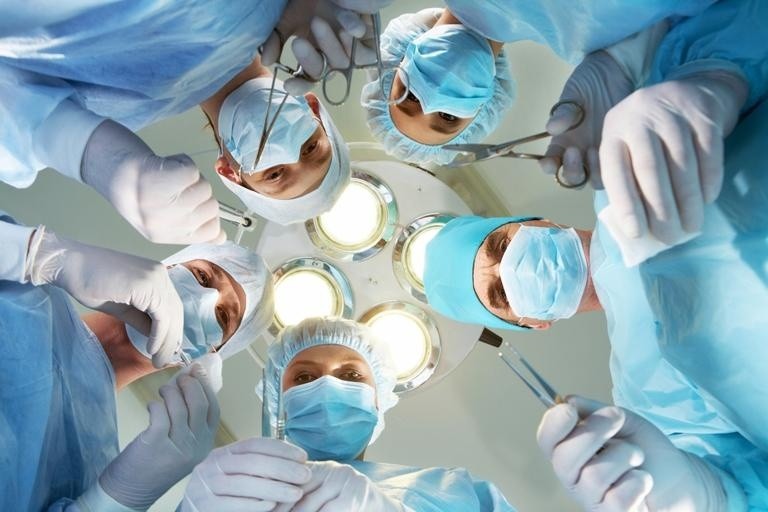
176;314;516;511
1;2;381;244
359;1;767;246
425;0;768;511
1;211;276;497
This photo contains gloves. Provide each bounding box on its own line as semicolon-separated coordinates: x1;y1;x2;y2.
180;438;404;512
542;51;637;191
598;69;748;244
98;362;220;512
26;224;184;368
536;394;725;512
81;119;227;246
261;0;392;96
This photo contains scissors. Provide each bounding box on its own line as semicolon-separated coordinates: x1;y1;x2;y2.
323;13;409;105
442;99;590;189
253;26;329;167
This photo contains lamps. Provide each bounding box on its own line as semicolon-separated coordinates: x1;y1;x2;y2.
224;140;486;406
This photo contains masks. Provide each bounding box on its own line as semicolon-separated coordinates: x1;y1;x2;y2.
125;263;223;364
283;375;380;461
399;24;496;118
218;78;321;181
499;219;588;327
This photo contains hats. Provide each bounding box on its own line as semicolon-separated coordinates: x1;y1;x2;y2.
423;216;543;331
